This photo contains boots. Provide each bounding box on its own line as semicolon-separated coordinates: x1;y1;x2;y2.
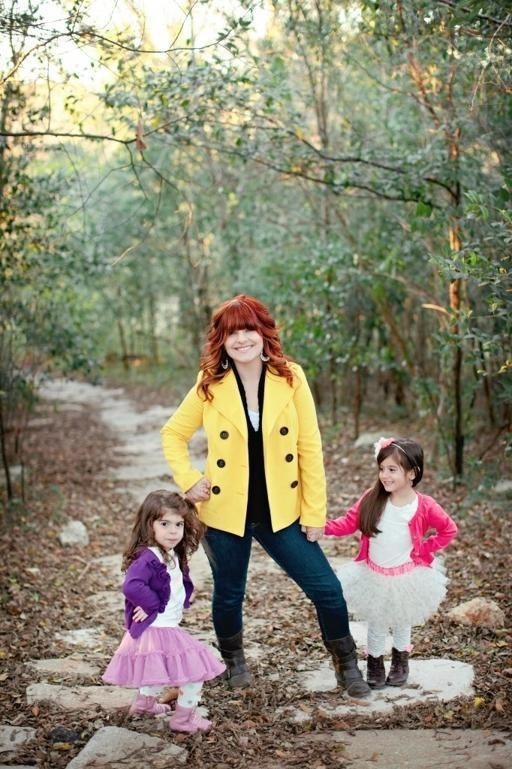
386;646;409;686
367;653;386;689
322;635;371;697
217;631;252;688
129;692;173;716
169;704;211;733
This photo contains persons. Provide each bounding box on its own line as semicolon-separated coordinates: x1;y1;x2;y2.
301;436;458;690
101;484;226;733
159;291;372;698
